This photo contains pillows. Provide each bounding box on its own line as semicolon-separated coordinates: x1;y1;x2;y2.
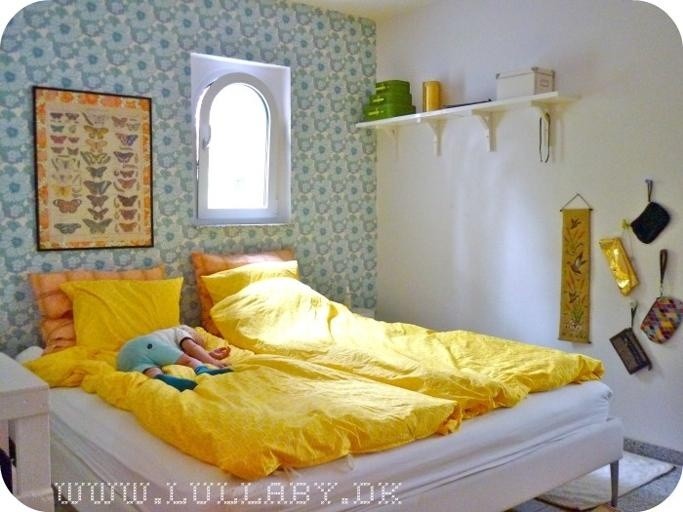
27;251;301;347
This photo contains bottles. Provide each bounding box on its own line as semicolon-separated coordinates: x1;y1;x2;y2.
423;81;441;111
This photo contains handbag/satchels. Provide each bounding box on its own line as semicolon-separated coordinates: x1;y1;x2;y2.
609;300;652;375
600;218;638;296
641;249;681;346
631;178;670;243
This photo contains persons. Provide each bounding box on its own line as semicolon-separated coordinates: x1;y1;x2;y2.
117;325;235;391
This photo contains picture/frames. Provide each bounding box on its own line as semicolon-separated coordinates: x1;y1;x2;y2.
31;85;154;250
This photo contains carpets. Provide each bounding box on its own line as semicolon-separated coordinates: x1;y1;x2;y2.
533;450;676;509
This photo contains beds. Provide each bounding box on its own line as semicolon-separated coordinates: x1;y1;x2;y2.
16;314;622;511
